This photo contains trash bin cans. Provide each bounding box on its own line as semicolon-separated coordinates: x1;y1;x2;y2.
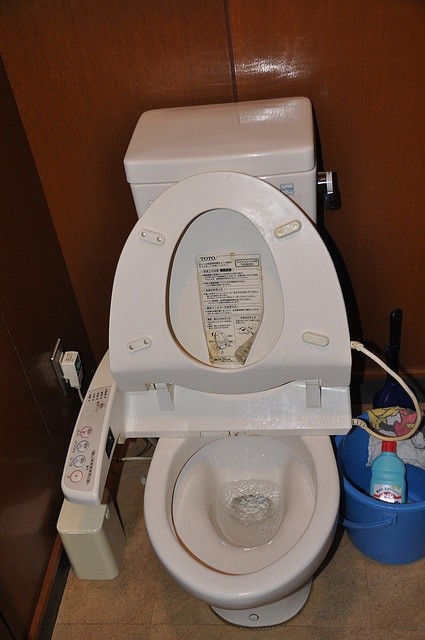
55;488;128;580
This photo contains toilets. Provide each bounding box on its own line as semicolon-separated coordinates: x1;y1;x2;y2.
107;94;355;629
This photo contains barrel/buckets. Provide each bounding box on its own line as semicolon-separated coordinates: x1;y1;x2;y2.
333;407;425;564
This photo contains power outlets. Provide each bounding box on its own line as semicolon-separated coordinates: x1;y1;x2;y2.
51;338;71;396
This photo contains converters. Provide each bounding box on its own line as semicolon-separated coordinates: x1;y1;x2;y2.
59;351;84;389
